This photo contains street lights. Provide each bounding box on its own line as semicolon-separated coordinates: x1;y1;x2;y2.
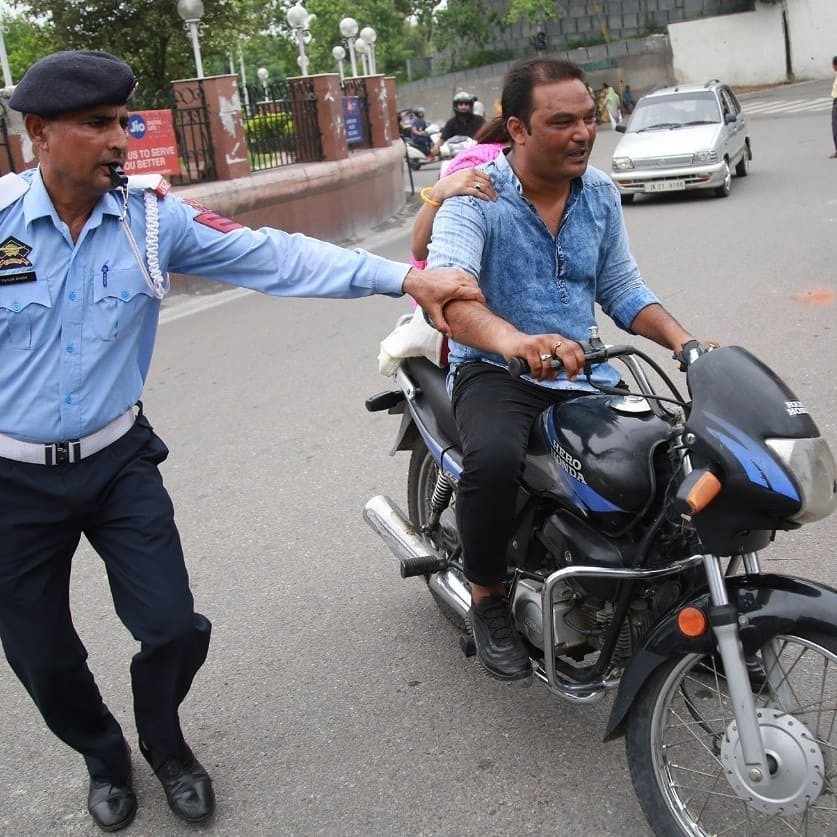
333;46;346;88
177;0;206;78
257;68;269;101
286;6;310;76
356;27;377;75
339;17;358;77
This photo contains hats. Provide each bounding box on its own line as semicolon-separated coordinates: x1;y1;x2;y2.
6;49;138;114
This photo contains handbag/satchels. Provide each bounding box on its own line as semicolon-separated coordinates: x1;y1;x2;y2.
617;108;622;123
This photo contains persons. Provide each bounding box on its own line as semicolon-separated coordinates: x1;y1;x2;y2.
408;116;516;366
411;108;435;160
0;51;485;829
830;56;837;158
429;92;488;157
583;81;636;130
426;61;718;681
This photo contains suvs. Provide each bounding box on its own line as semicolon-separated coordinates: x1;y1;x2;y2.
611;79;752;205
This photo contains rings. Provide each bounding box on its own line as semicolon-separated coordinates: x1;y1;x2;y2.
540;355;552;361
475;182;481;190
552;341;562;355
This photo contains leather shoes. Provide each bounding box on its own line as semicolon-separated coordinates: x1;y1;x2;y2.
88;742;136;833
138;735;216;822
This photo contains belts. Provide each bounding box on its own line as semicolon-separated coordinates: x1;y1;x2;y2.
0;406;136;467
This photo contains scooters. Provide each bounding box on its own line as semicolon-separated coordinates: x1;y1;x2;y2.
434;135;479;180
406;124;441;170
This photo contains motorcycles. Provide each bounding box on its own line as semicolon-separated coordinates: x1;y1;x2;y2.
362;314;837;837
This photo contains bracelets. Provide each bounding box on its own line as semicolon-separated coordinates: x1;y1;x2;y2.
420;188;442;207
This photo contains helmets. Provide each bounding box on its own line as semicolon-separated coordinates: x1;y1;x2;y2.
453;91;473;115
415;107;424;118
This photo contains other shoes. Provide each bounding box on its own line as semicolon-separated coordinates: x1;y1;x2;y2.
426;153;434;160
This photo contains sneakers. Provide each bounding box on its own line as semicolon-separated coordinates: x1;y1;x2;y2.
470;591;533;681
